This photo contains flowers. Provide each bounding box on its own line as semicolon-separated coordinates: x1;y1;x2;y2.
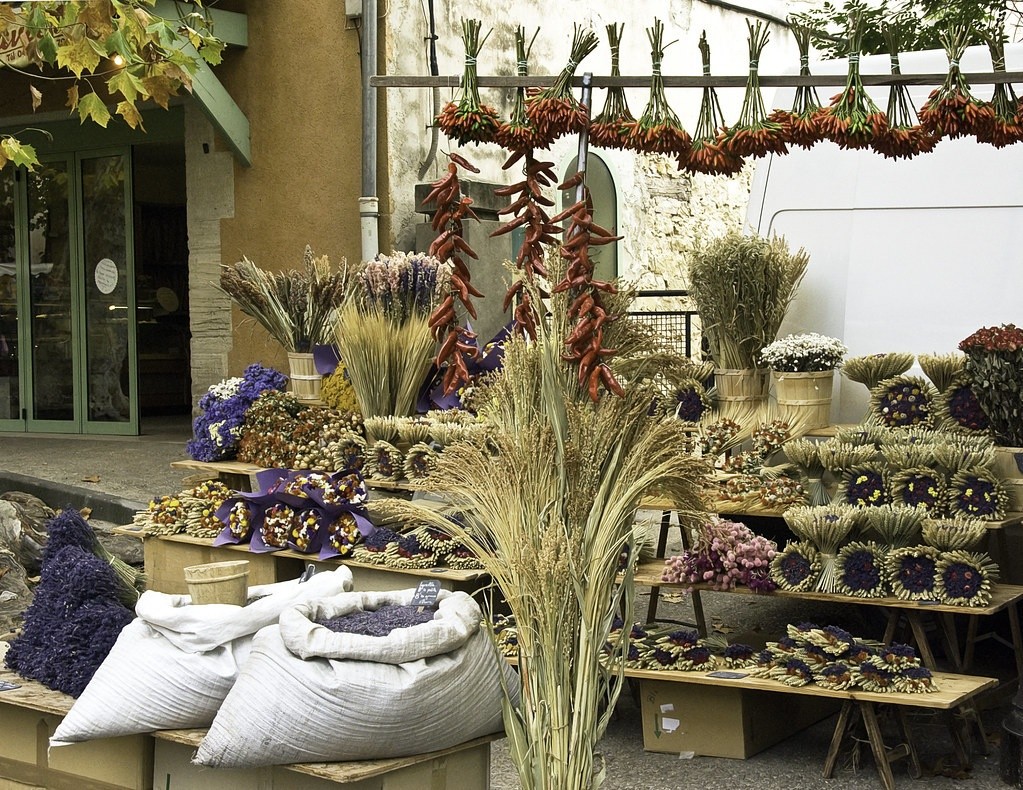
148;362;1007;606
757;330;850;392
956;323;1023;448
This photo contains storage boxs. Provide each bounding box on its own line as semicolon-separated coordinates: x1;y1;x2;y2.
152;739;491;790
303;559;459;593
639;630;842;761
142;536;291;595
0;703;154;790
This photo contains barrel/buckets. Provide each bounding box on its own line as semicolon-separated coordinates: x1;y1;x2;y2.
772;370;834;429
712;369;769;417
287;352;323;399
184;560;250;607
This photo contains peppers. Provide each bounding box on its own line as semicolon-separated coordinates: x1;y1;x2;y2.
420;146;628;402
431;86;1023;178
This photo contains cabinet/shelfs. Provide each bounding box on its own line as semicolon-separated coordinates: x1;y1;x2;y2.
111;419;1023;790
